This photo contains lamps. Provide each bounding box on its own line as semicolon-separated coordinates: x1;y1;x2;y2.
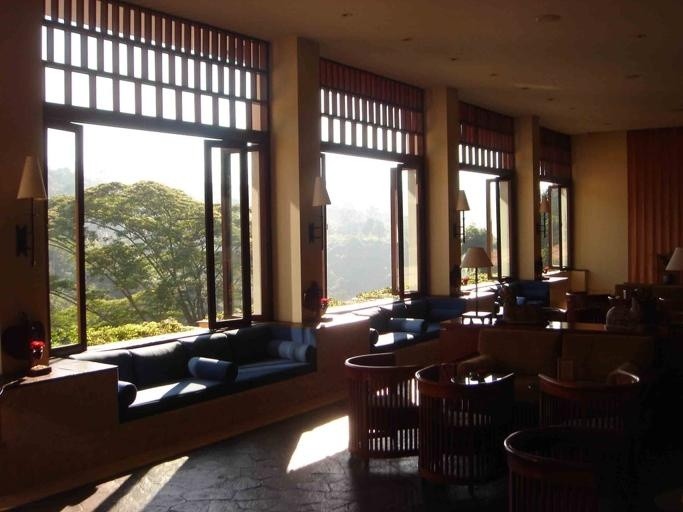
537;198;549;238
452;188;469;244
460;247;494;315
16;151;49;267
308;175;331;250
665;247;683;284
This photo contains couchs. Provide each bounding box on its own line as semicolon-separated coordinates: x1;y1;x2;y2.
71;323;319;422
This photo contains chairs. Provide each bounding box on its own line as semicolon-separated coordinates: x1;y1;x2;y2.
536;371;641;474
415;363;515;493
344;352;420;466
342;281;681;381
504;427;598;512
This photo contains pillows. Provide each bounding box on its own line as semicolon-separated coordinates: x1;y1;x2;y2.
186;356;238;382
265;339;312;360
116;379;137;407
